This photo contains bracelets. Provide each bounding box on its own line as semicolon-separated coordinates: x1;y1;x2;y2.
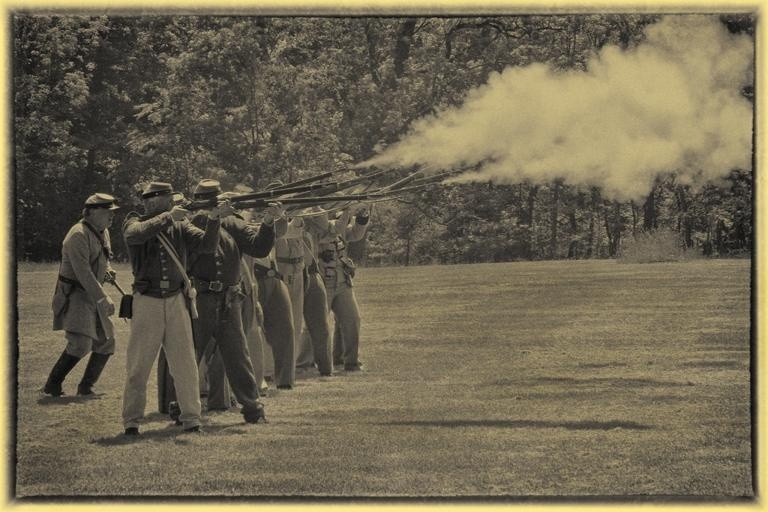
94;294;107;304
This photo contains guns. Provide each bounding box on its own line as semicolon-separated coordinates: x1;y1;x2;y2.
182;163;479;211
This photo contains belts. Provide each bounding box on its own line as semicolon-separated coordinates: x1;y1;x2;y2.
151;281;177;289
198;281;232;293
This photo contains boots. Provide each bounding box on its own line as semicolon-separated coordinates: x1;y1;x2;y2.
77;352;111;395
43;349;82;396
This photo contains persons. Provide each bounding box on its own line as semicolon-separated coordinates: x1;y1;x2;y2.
43;191;116;397
169;178;283;425
207;178;373;409
121;183;229;436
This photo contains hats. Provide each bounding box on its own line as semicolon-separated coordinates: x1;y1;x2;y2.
84;192;120;211
194;179;221;196
263;179;283;191
171;192;192;207
234;183;254;194
141;181;179;199
